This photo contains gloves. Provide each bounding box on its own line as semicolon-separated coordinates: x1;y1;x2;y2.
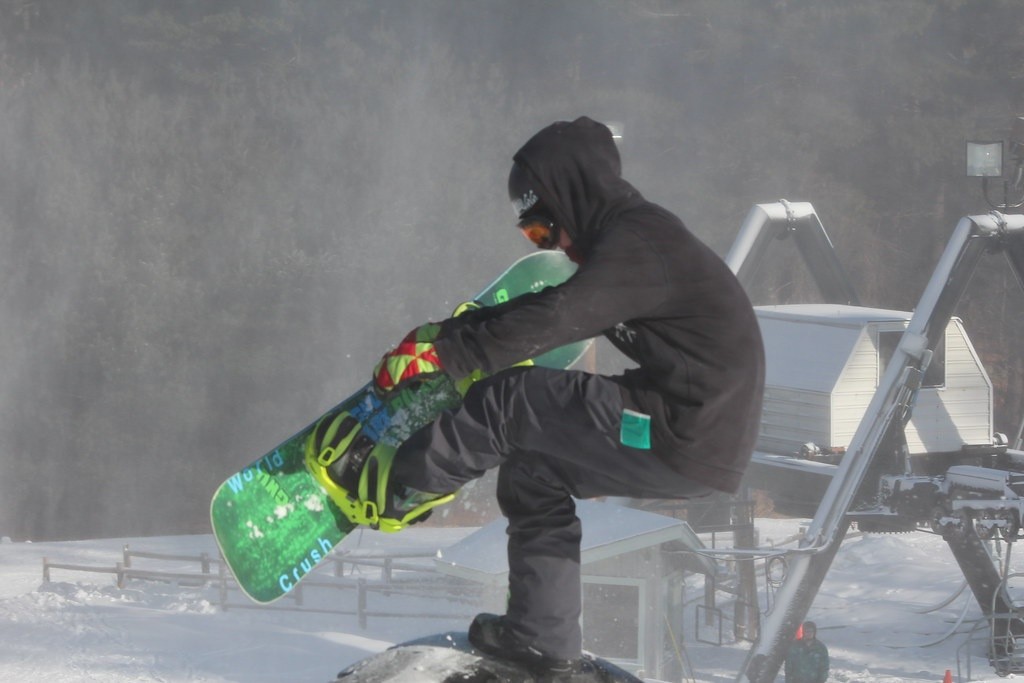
371;322;444;393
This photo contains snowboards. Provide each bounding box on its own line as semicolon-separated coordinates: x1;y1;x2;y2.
208;245;598;609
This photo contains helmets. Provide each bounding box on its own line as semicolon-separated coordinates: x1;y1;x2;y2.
508;163;545;218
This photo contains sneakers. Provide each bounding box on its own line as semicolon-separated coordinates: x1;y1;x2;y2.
315;411;438;524
470;611;574;679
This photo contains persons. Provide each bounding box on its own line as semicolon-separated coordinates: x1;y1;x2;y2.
785;621;829;683
305;116;766;674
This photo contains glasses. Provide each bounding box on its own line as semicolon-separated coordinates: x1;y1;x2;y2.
516;215;559;250
